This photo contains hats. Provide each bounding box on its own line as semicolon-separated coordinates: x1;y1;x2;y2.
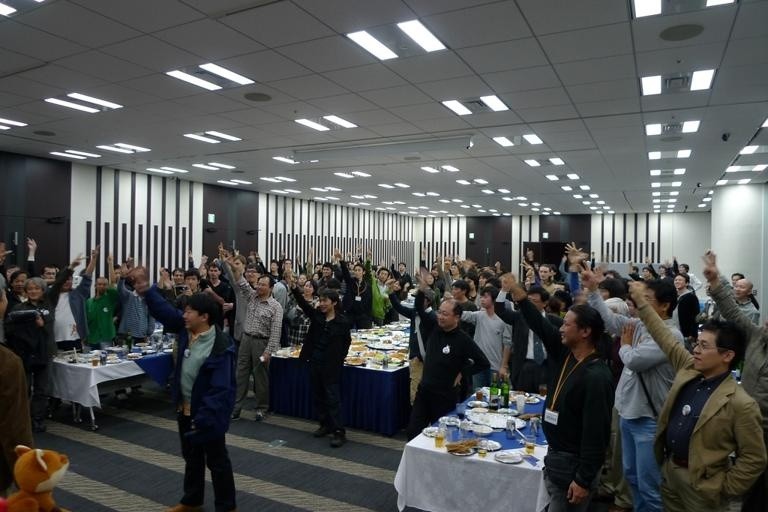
411;290;433;302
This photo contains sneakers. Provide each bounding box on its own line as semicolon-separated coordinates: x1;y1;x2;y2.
255;410;267;421
312;426;337;438
31;421;42;432
330;434;346;448
164;502;202;511
230;414;241;421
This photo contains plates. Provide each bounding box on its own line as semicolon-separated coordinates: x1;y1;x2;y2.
466;391;540;429
107;343;156;363
276;347;302;358
344;322;411;366
452;440;523;464
423;416;472;437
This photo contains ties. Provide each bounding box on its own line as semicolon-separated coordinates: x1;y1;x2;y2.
532;331;544;366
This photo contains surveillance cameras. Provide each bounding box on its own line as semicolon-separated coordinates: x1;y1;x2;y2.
466;138;473;150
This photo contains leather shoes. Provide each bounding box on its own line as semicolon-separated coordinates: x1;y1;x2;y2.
130;384;142;389
131;389;144;395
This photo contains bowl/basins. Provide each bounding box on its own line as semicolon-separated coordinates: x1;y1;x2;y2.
474;426;493;437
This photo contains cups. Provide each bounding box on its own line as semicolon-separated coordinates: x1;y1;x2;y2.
456;403;465;419
93;355;98;366
435;430;444;448
539;384;547;397
478;438;487;455
525;435;535;456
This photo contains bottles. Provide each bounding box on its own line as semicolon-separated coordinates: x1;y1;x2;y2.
699;296;704;311
100;352;106;365
383;355;388;369
127;331;132;352
489;373;509;407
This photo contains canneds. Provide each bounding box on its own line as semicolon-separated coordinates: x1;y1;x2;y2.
506;419;516;439
530;417;539;436
156;343;162;353
100;352;106;365
383;358;388;369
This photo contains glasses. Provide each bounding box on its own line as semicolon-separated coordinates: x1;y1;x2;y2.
691;342;728;353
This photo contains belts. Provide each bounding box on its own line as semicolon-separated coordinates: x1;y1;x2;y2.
244;331;269;339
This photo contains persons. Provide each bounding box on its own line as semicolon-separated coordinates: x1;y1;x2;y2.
386;240;768;512
0;242;414;512
